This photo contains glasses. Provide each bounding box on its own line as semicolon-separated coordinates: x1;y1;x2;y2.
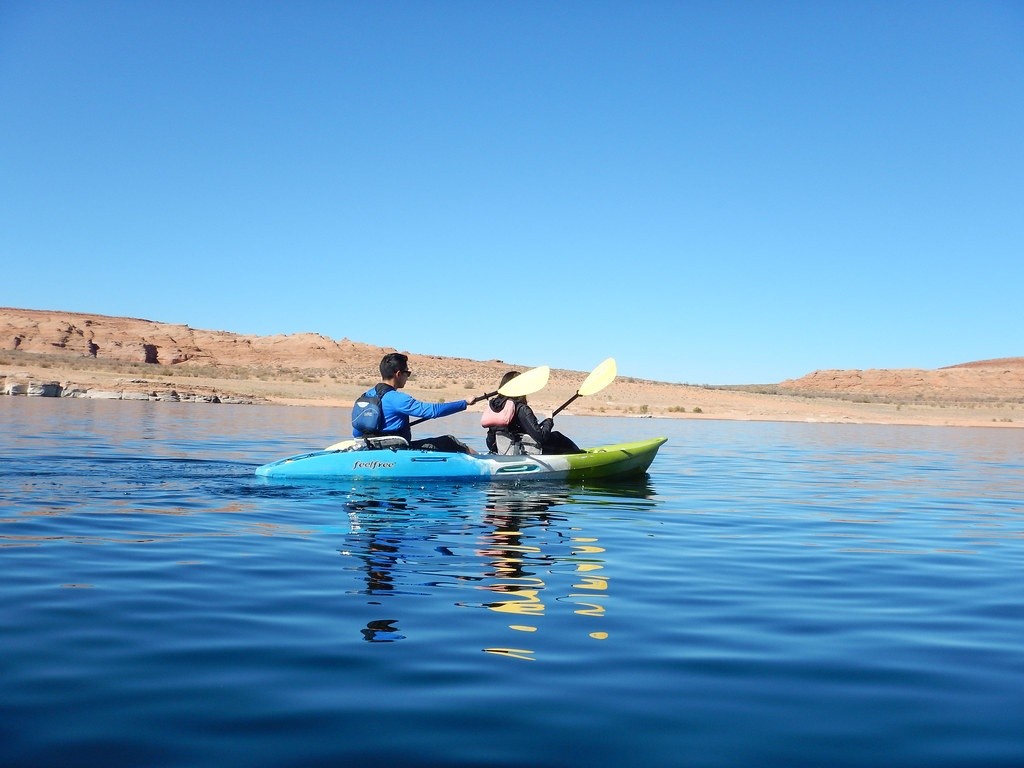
394;371;411;377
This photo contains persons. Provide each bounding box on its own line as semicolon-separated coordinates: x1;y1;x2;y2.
480;371;607;455
351;353;479;454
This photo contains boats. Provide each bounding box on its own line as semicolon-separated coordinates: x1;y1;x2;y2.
254;438;667;480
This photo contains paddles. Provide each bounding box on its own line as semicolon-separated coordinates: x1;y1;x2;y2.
539;357;617;422
324;367;550;451
